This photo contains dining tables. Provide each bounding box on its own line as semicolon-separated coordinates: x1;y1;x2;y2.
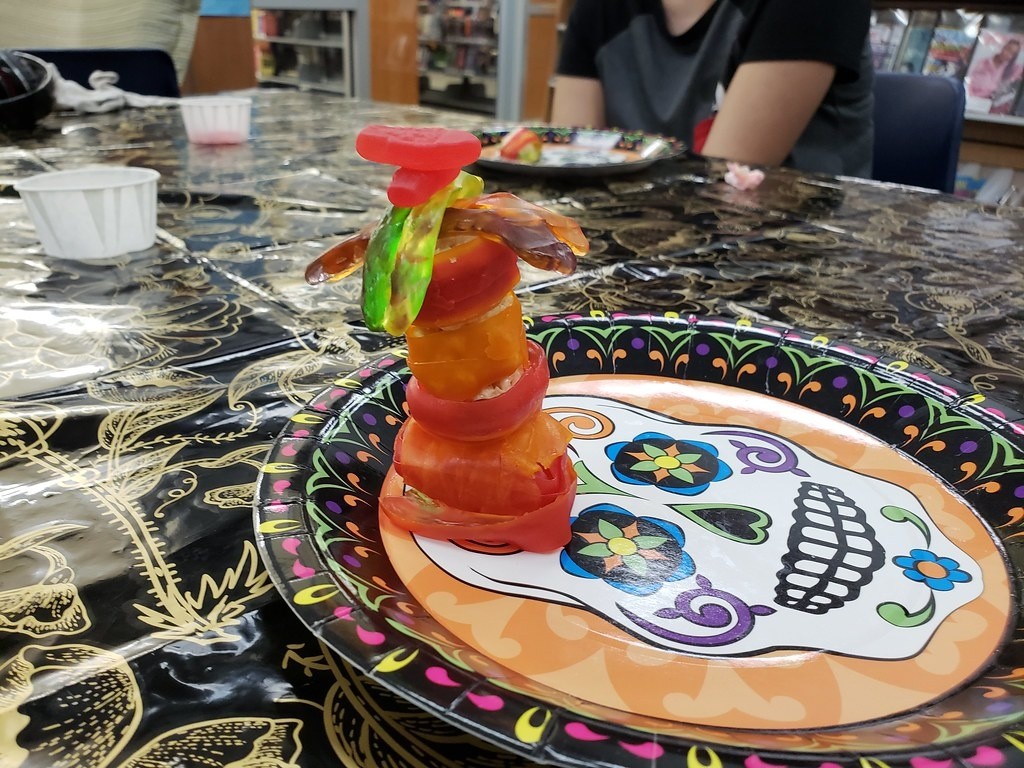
2;90;1024;768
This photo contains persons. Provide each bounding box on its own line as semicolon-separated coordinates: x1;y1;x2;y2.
550;2;879;179
968;39;1023;99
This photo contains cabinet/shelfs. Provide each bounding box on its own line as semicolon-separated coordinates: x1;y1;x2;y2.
251;1;355;108
417;0;500;116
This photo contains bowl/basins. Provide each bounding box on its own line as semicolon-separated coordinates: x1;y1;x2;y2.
11;164;161;260
180;92;251;146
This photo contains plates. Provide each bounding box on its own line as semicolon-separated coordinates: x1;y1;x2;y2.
251;312;1022;766
470;123;691;172
